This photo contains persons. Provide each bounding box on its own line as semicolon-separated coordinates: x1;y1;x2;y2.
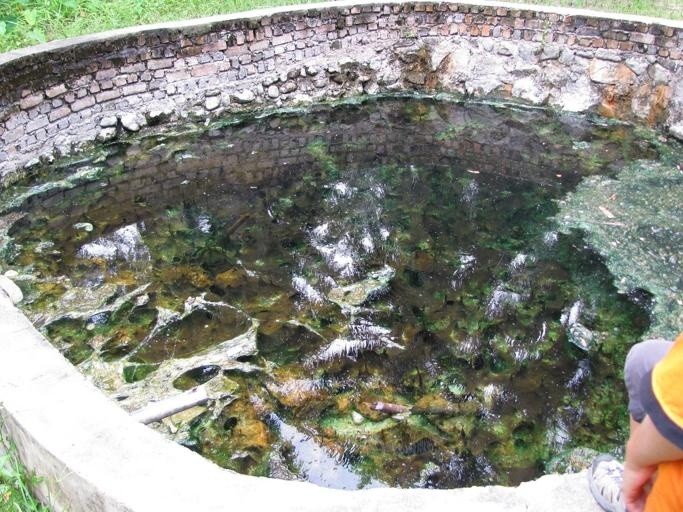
589;333;683;512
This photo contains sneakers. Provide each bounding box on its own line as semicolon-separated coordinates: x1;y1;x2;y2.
589;453;624;512
